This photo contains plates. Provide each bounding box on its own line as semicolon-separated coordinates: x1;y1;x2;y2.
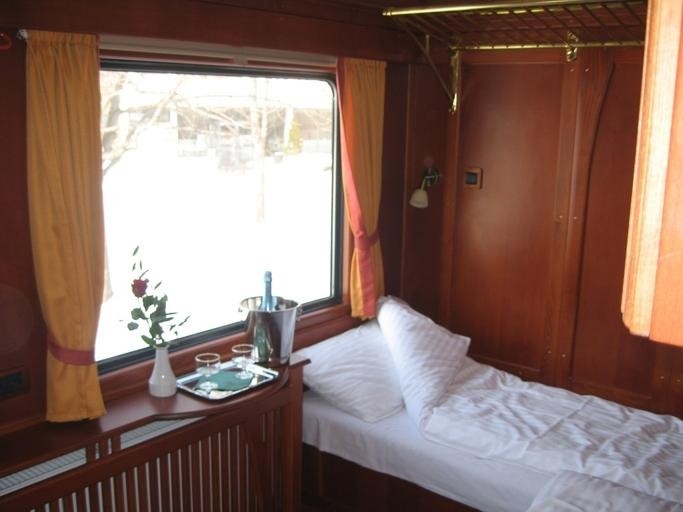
176;358;280;403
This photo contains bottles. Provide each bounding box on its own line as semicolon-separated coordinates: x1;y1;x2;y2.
259;271;276;311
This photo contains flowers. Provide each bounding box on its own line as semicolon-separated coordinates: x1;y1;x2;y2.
125;247;191;349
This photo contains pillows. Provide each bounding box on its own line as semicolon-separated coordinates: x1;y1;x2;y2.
294;322;404;424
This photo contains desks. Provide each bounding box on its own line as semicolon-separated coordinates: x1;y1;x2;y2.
0;348;312;511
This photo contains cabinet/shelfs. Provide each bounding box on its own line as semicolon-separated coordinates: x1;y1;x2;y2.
441;43;683;418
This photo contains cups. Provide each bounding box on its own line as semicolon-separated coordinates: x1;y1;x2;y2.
232;344;256;380
194;352;221;391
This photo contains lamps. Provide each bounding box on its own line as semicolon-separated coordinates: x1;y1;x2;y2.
408;166;437;208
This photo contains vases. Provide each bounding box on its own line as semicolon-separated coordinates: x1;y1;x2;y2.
148;349;179;399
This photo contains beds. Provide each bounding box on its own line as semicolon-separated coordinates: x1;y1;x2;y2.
294;314;683;512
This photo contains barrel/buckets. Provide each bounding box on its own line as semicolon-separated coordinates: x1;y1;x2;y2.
239;296;299;366
